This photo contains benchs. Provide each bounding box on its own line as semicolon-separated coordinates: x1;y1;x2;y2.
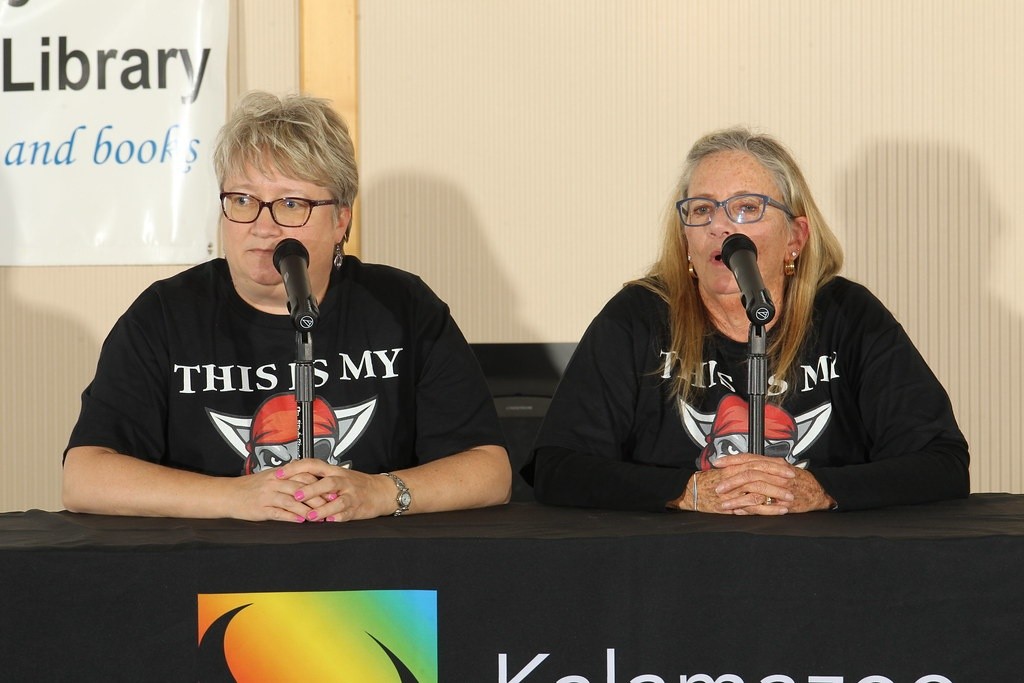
468;342;583;501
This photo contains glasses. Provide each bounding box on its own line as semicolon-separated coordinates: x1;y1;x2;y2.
219;185;339;228
676;193;792;227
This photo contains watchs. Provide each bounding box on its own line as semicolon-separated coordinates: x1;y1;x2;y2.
380;471;412;517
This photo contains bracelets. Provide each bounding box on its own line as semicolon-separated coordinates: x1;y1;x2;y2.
694;470;705;512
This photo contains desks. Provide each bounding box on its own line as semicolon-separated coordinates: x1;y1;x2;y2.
0;492;1024;683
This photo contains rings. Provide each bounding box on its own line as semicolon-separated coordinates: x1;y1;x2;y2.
766;495;772;506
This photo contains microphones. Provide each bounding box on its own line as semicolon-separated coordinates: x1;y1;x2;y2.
722;233;775;326
272;238;320;332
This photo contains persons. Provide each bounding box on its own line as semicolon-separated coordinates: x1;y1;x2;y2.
59;95;514;523
531;128;972;517
